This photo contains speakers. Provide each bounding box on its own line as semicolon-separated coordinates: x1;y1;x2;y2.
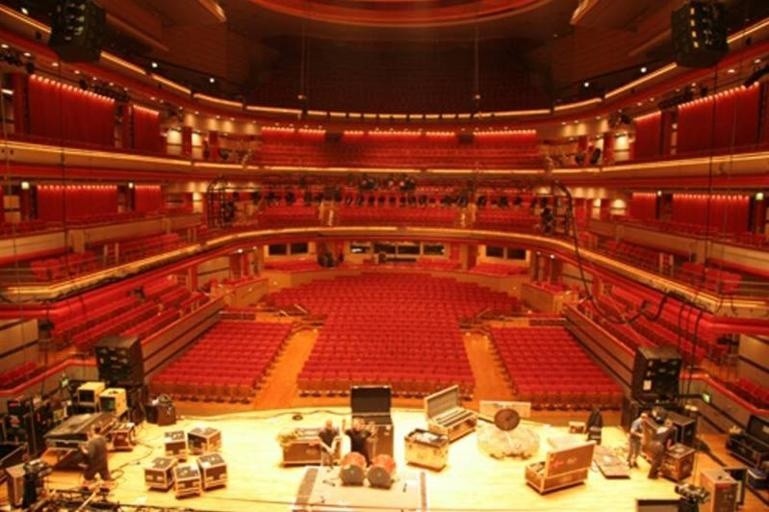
670;3;729;71
49;3;106;63
632;347;683;401
96;337;144;386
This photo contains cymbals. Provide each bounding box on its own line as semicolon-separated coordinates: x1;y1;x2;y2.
494;409;519;431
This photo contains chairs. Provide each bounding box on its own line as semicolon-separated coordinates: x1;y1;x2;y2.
0;142;765;415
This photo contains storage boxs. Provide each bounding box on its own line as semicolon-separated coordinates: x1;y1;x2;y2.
424;383;477;443
524;439;595;494
700;468;739;512
144;426;228;498
349;384;394;457
403;427;450;472
281;428;342;466
660;442;696;483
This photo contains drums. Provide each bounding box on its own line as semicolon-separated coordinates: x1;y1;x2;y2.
339;453;367;485
368;454;396;487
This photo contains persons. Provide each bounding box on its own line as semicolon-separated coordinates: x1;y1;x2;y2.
648;420;673;478
319;419;339;472
342;416;372;466
585;403;604;444
628;413;647;468
77;422;110;483
